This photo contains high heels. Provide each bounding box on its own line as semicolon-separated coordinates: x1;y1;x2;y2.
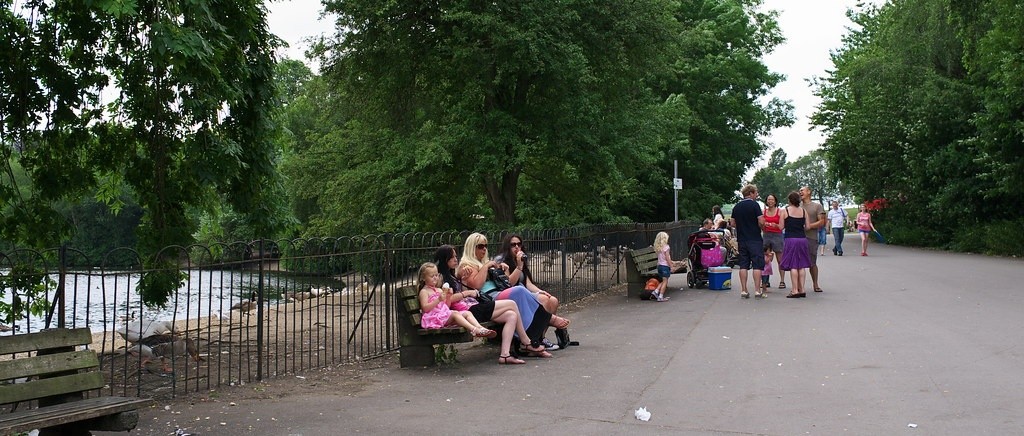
798;289;806;298
786;291;800;298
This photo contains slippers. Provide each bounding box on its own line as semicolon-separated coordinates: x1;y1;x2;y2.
813;287;823;292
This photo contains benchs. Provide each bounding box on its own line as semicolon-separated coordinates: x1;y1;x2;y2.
625;247;658;297
0;328;154;436
395;286;497;367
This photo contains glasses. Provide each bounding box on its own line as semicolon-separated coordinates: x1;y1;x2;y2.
476;243;490;249
509;242;523;248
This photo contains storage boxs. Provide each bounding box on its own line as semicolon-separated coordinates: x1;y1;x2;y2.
708;267;732;291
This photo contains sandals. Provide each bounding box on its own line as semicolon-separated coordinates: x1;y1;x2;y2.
472;328;491;336
520;342;545;352
549;314;570;329
483;329;496;338
761;283;770;288
779;282;786;288
530;351;552;358
499;356;524;364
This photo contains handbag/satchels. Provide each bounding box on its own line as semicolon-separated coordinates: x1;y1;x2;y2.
475;290;493;304
489;267;512;291
701;245;723;268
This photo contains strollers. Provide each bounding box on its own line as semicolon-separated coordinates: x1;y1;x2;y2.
686;229;729;290
723;237;753;270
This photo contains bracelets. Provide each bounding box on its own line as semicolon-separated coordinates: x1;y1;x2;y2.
460;291;465;298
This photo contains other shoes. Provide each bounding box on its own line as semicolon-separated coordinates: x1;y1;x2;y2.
838;251;842;256
740;291;750;299
651;291;659;299
763;289;770;292
862;252;868;257
755;291;768;298
833;247;837;255
657;297;668;302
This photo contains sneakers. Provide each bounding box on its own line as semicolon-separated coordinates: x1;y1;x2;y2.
542;338;559;350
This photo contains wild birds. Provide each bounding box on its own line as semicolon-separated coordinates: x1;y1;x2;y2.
214;310;229;323
280;285;338;302
229;291;257;317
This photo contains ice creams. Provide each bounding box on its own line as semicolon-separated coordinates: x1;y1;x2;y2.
442;282;449;293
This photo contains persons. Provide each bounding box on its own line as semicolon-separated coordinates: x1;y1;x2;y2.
713;205;725;225
848;216;857;231
456;233;571;358
819;226;826;256
762;193;786;289
798;186;826;292
731;184;765;299
762;245;774;293
856;204;878;256
826;201;850;256
779;192;810;298
651;232;676;302
717;219;731;238
433;245;545;364
416;262;497;338
699;219;727;265
493;234;559;351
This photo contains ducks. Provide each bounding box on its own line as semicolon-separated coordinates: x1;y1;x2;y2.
117;317;181;354
127;334;199;372
542;244;631;269
120;311;135;321
